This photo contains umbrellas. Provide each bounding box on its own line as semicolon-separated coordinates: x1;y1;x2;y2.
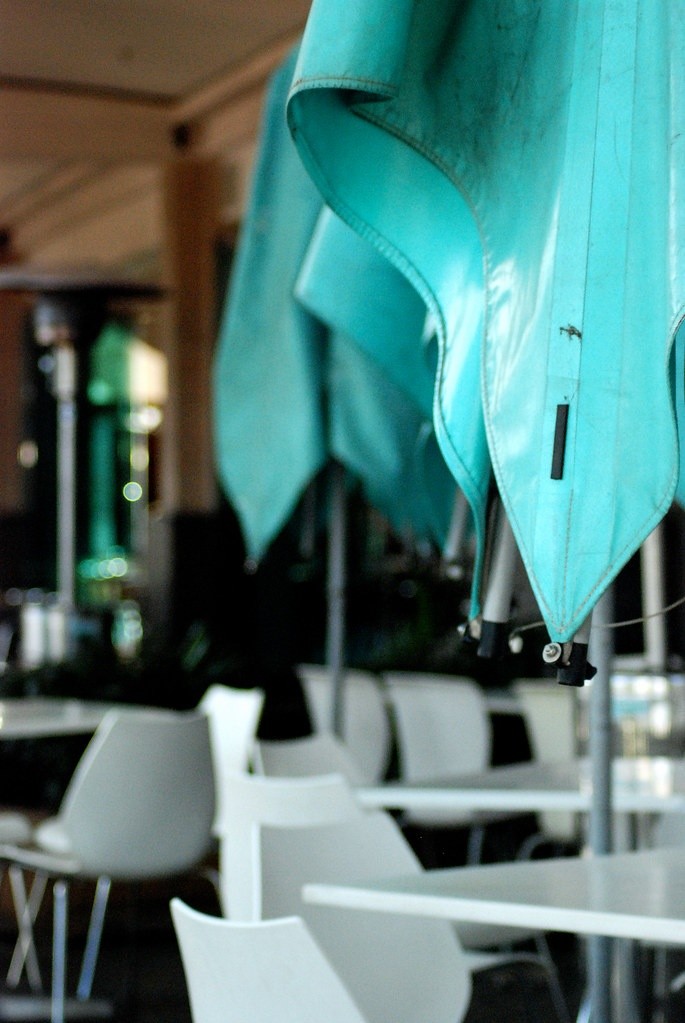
209;0;685;1023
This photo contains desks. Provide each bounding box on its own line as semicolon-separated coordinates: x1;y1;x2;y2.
301;847;685;1023
373;754;685;849
2;696;167;742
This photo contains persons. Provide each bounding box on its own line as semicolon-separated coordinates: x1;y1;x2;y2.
129;504;308;722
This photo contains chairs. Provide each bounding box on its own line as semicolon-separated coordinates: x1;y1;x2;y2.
2;664;685;1022
